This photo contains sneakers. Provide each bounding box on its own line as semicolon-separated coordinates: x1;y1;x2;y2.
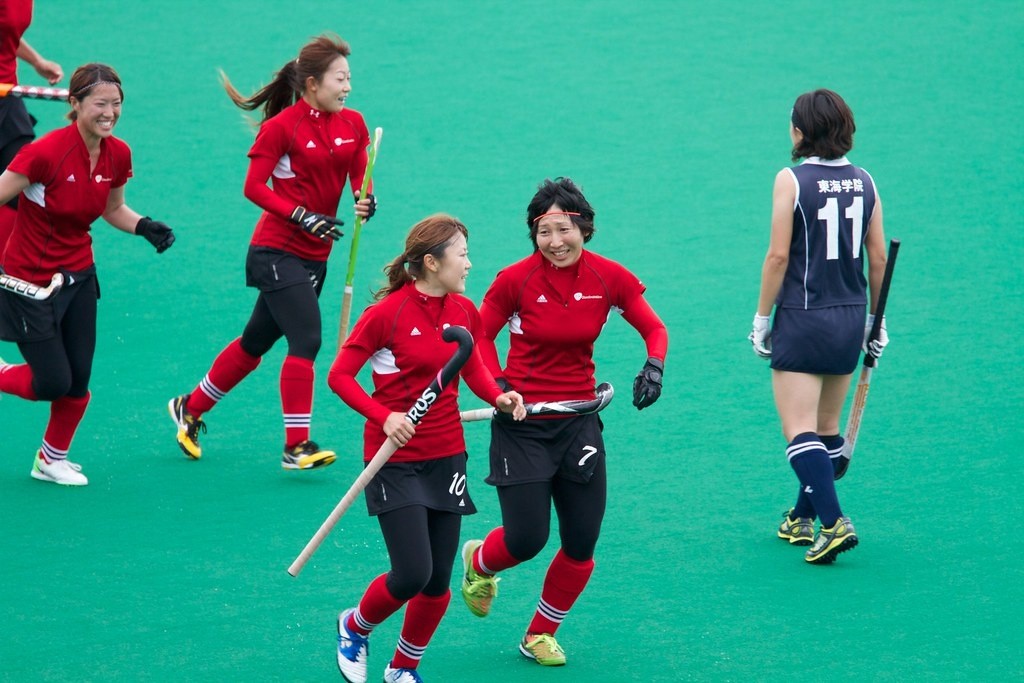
168;394;203;460
519;633;566;666
462;540;501;618
336;608;369;683
778;508;815;545
30;449;88;487
280;440;337;470
804;516;859;564
383;660;423;683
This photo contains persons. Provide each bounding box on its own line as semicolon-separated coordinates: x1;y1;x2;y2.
0;0;176;485
462;175;668;666
750;87;890;562
327;210;526;683
167;30;378;470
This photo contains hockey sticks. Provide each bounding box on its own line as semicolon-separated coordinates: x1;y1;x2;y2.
0;83;70;103
286;326;475;576
337;127;383;355
832;238;901;480
0;270;64;302
458;382;614;422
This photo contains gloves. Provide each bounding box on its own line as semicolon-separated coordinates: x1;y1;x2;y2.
632;357;664;410
291;205;346;243
861;313;887;369
491;379;523;426
747;312;774;359
135;217;175;254
